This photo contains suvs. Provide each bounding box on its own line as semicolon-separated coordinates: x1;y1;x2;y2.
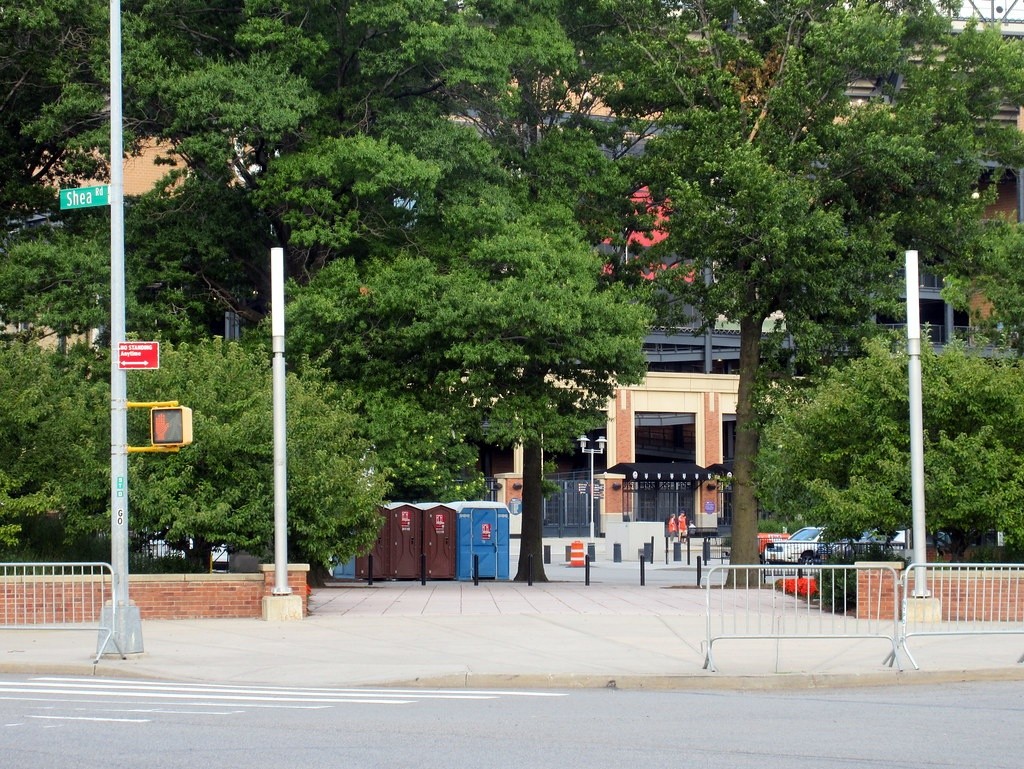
766;526;829;565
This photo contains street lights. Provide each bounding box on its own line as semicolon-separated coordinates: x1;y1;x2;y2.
578;434;607;537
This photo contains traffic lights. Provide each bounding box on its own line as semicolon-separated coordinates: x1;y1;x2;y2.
150;405;193;446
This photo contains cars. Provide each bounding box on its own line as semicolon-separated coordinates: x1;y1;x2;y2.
816;528;950;565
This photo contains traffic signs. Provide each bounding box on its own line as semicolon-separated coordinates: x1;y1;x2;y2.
59;184;110;209
117;341;159;369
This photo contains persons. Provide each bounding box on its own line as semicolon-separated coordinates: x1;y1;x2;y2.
678;510;687;544
684;520;696;543
668;513;678;544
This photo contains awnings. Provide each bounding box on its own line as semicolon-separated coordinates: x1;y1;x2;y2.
603;462;735;492
